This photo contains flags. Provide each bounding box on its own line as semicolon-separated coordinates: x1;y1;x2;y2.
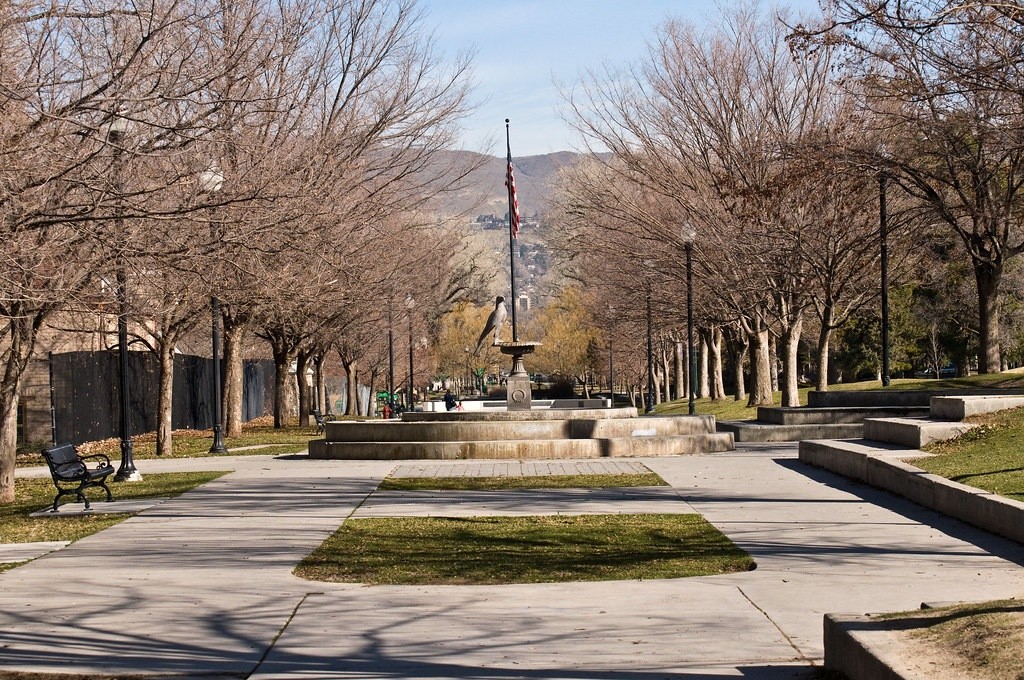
505;140;521;239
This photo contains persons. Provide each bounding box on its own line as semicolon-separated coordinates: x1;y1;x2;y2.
383;389;464;419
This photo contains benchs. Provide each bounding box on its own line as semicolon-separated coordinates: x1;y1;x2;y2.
40;443;115;513
312;410;336;435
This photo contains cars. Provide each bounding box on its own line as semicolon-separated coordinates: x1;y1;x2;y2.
940;363;957;377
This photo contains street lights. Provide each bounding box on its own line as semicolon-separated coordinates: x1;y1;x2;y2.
405;291;417;412
681;215;697;416
871;143;894;388
606;304;616;406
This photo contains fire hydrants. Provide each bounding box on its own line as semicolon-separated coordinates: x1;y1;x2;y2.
382;405;393;419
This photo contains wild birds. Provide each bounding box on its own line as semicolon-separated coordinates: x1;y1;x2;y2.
473;294;508;357
